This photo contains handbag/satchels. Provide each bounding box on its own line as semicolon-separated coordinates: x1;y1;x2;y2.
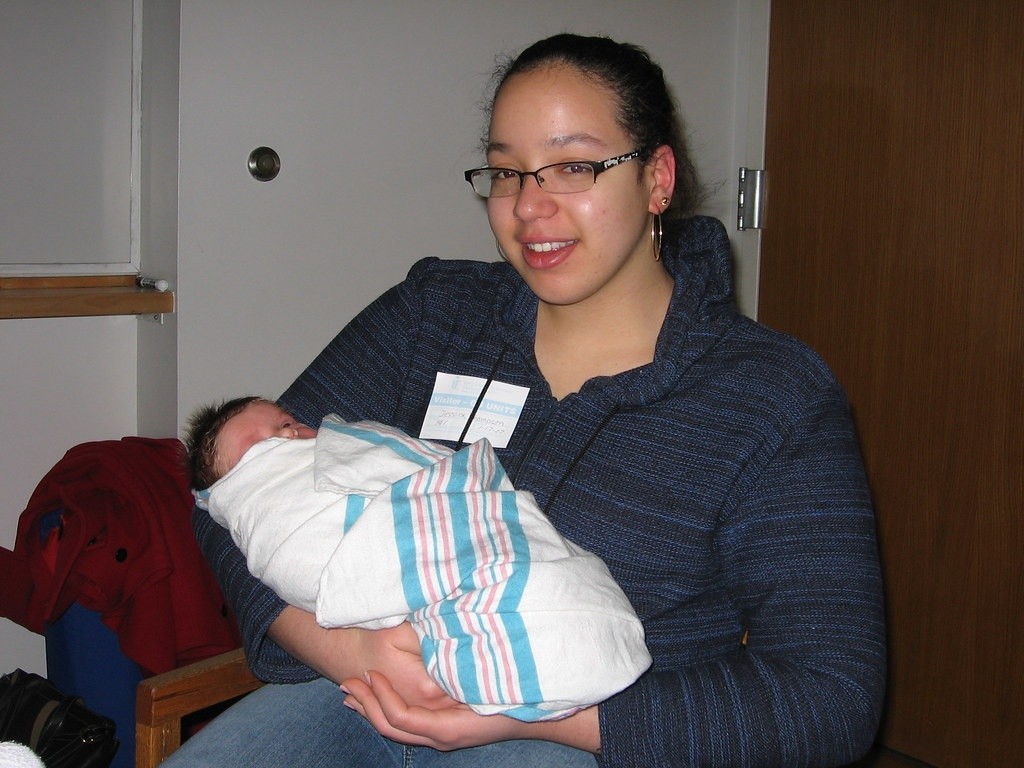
0;668;121;768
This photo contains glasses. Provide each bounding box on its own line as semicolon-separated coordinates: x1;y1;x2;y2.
465;142;663;199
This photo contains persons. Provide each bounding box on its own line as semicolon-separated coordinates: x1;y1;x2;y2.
160;32;890;767
177;393;653;725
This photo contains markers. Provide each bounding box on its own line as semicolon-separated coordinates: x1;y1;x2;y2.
134;276;170;292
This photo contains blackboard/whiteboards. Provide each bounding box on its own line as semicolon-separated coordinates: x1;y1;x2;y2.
0;0;144;277
